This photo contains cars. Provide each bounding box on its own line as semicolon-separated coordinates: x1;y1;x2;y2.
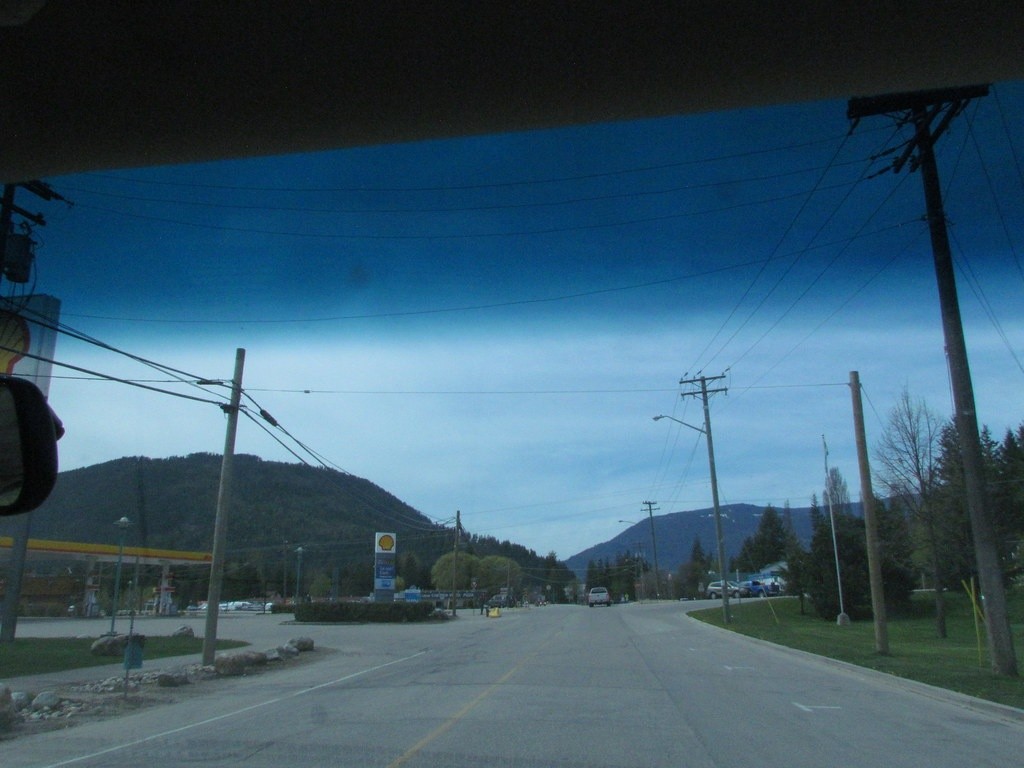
484;593;548;608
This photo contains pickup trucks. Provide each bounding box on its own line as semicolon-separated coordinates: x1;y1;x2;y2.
588;587;610;607
739;580;779;598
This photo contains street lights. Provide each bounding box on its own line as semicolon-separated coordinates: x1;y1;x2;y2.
653;414;731;625
293;547;303;616
104;518;134;637
619;520;661;601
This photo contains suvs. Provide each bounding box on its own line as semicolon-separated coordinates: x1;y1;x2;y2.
707;581;751;598
